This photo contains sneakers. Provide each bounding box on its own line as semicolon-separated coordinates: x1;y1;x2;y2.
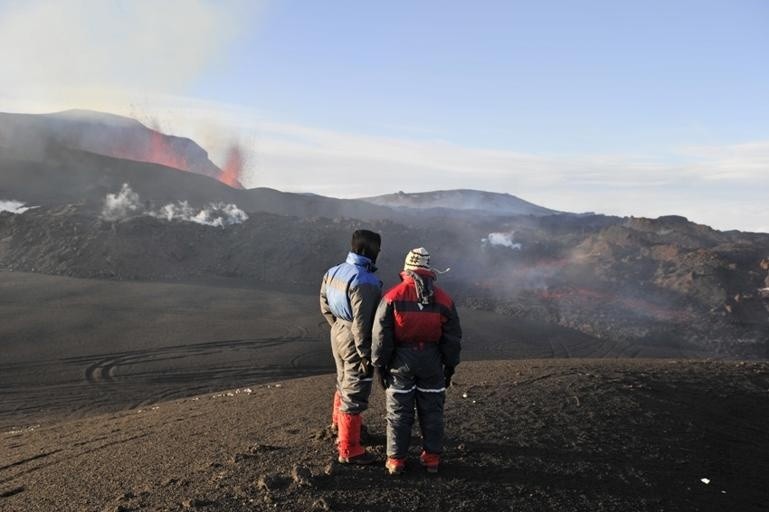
331;421;369;435
339;449;377;465
385;457;406;476
419;450;440;474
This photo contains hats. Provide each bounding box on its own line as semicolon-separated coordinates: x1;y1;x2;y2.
403;246;432;273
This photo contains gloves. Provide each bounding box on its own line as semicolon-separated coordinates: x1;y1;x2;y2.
357;359;374;379
371;367;392;390
443;368;454;388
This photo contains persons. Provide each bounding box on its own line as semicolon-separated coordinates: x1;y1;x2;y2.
368;245;463;477
320;229;383;464
